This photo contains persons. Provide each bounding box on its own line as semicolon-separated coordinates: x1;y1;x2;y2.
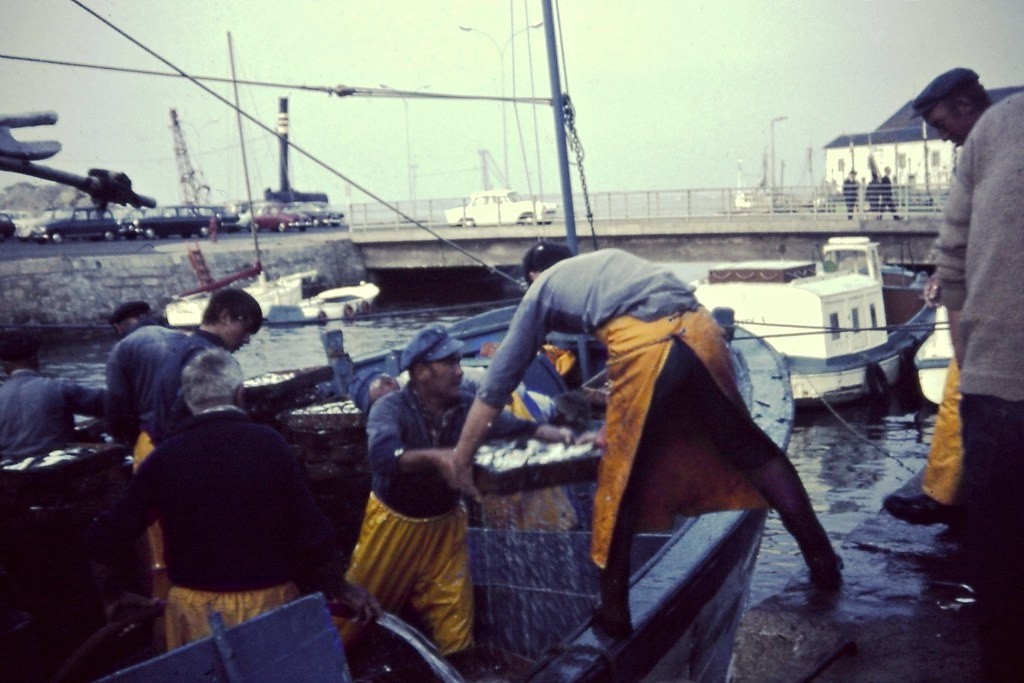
80;346;377;659
937;89;1023;683
328;325;575;683
883;67;993;525
100;288;263;653
840;166;903;221
1;331;106;460
450;243;844;636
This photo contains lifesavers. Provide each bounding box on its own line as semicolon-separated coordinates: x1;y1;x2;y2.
318;311;329;322
344;305;354;318
866;362;892;408
362;302;369;314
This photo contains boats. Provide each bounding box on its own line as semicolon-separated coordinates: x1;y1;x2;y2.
694;237;954;408
0;292;794;683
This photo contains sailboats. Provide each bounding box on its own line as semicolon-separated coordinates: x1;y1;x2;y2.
162;28;379;326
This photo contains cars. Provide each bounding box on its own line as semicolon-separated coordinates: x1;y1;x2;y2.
211;202;251;233
296;200;344;228
117;205;213;240
0;207;119;244
247;204;312;232
446;190;558;228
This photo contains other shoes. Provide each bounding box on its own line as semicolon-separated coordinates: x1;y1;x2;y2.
591;607;635;640
884;494;955;526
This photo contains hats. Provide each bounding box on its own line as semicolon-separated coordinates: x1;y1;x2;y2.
0;331;41;361
109;302;149;324
910;68;979;117
400;325;464;370
712;309;738;328
